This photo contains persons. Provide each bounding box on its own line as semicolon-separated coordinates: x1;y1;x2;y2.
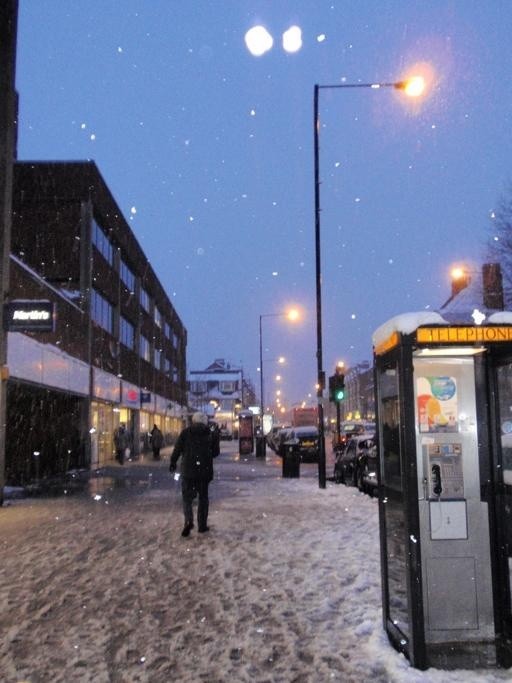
150;424;164;461
169;412;220;537
214;425;221;434
114;423;131;465
381;395;401;477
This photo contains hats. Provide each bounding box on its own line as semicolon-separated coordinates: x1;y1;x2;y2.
191;412;209;425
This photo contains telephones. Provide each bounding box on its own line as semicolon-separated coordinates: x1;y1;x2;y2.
423;444;465;500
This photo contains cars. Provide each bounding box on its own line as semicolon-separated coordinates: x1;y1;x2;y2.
268;426;318;463
334;423;378;497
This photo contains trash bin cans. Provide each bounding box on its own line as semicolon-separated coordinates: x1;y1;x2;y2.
256;435;266;457
282;442;301;477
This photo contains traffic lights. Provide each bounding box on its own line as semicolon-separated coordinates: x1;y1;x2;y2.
329;374;346;403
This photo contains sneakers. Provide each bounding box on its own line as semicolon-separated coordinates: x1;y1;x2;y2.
181;521;194;536
198;526;209;533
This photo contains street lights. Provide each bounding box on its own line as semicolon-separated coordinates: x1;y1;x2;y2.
440;268;481;309
314;76;425;488
262;357;285;408
260;310;300;459
